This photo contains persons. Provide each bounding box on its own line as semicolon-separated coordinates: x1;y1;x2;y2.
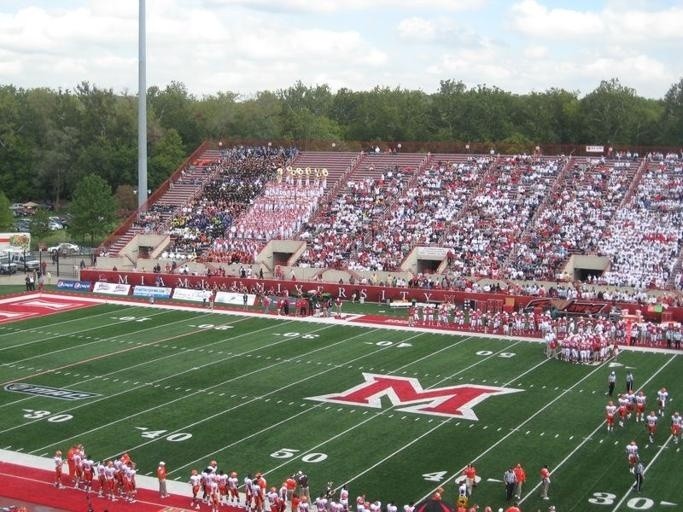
190;461;266;511
81;260;85;270
112;266;117;271
407;303;683;364
432;143;683;306
268;471;349;511
53;444;137;504
152;275;432;318
540;465;550;499
24;253;58;292
356;495;398;511
157;462;170;498
606;371;683;492
403;501;416;511
132;143;432;279
457;462;526;511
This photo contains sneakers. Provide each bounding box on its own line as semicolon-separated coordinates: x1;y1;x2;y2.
191;500;200;509
97;492;137;504
73;483;91;493
507;495;521;500
161;493;170;499
202;497;243;511
658;412;665;417
52;482;66;489
540;494;550;499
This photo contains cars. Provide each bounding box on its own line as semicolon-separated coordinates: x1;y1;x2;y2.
8;203;54;218
9;215;70;231
48;242;78;254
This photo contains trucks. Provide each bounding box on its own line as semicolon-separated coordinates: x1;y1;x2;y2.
0;247;40;274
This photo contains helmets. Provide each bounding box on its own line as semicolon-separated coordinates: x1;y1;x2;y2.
55;444;136;476
608;390;644;405
649;410;656;417
673;412;680;417
191;460;238;478
159;461;166;466
272;470;303;493
660;387;667;393
